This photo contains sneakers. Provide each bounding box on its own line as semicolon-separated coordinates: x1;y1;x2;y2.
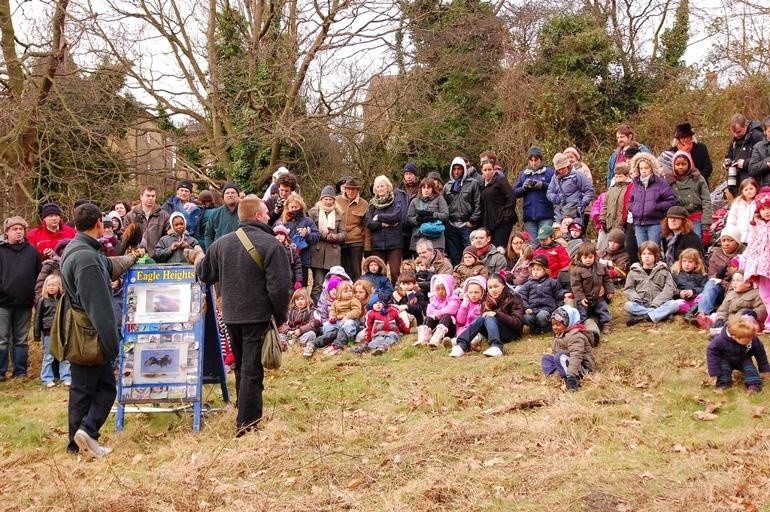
303;348;312;358
443;337;451;351
350;344;368;354
64;382;71;387
323;346;341;356
73;429;103;461
47;382;56;388
448;346;464;358
100;447;115;454
372;348;385;357
603;324;610;335
626;316;642;327
483;347;502;357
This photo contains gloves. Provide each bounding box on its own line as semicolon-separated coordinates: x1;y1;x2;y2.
522;178;531;190
742;310;758;319
701;230;712;246
566;378;576;391
329;316;337;324
416;209;434;216
712;319;726;328
531;180;542;189
417;216;431;223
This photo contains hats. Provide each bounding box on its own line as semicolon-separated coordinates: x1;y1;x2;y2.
198;189;216;203
754;191;770;211
3;216;28;232
41;203;61;219
550;307;571;328
722;224;742;246
673;123;695;137
569;223;582;232
273;226;288;236
403;162;418;176
328;277;341;290
342;176;362;188
176;180;193;193
563;146;581;160
434;279;443;288
529;254;548;269
622;148;640;157
102;216;114;226
608;228;625;244
553;153;570;170
367;291;387;309
270;184;279;193
320;185;336;199
527;146;542;158
222;183;240;197
428;171;443;181
566;239;584;261
667;205;688;219
730;255;739;270
462;245;478;259
536;225;554;239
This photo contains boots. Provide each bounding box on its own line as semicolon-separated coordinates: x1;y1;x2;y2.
427;323;448;350
414;324;432;347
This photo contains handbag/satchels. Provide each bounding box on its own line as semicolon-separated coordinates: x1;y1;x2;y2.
558;200;579;219
47;293;108;367
260;322;282;371
418;220;445;238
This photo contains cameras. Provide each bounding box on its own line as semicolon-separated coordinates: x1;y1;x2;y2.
275;197;287;209
722;157;741;189
528;179;537;186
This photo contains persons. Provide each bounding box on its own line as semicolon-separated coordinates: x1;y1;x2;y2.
7;115;769;459
